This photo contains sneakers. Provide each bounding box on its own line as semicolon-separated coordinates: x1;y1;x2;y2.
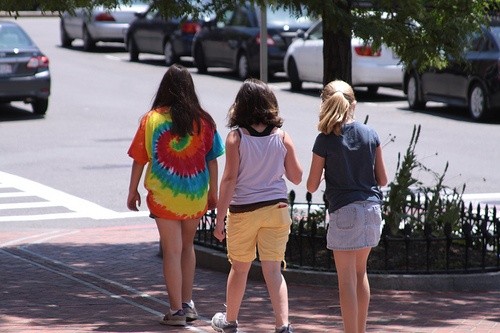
273;322;293;333
158;308;187;326
182;299;199;321
210;312;239;333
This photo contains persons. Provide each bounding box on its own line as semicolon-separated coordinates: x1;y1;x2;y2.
127;64;225;324
306;81;388;333
211;78;304;333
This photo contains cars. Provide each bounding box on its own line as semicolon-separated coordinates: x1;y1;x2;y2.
403;14;500;120
0;20;51;114
59;0;160;52
283;10;445;90
191;0;322;80
126;0;218;65
